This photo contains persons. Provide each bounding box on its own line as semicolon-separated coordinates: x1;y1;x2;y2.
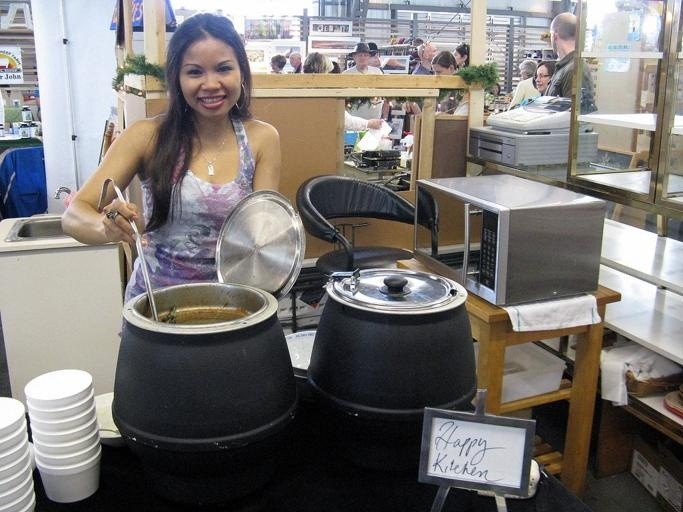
60;12;282;338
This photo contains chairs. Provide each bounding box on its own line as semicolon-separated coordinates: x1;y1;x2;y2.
290;174;416;334
416;186;440;260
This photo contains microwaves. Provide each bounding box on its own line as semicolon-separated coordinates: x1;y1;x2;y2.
413;171;609;307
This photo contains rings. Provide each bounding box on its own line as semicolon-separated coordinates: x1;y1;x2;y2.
104;210;118;225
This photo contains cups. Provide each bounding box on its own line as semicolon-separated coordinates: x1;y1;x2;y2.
0;368;101;512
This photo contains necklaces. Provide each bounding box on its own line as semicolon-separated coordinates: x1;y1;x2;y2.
193;129;231;176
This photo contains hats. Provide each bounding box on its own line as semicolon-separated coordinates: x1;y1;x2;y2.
349;42;378;58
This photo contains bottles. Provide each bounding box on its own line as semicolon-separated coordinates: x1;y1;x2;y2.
21;105;33;123
20;123;30;139
30;122;39;139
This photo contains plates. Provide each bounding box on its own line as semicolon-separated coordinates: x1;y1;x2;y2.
286;331;319;379
93;390;125;438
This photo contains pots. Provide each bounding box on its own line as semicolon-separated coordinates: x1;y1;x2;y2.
109;282;300;454
308;267;478;424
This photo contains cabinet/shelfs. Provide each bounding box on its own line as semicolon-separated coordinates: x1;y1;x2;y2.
463;155;683;480
566;0;683;236
394;258;622;505
356;1;559;100
